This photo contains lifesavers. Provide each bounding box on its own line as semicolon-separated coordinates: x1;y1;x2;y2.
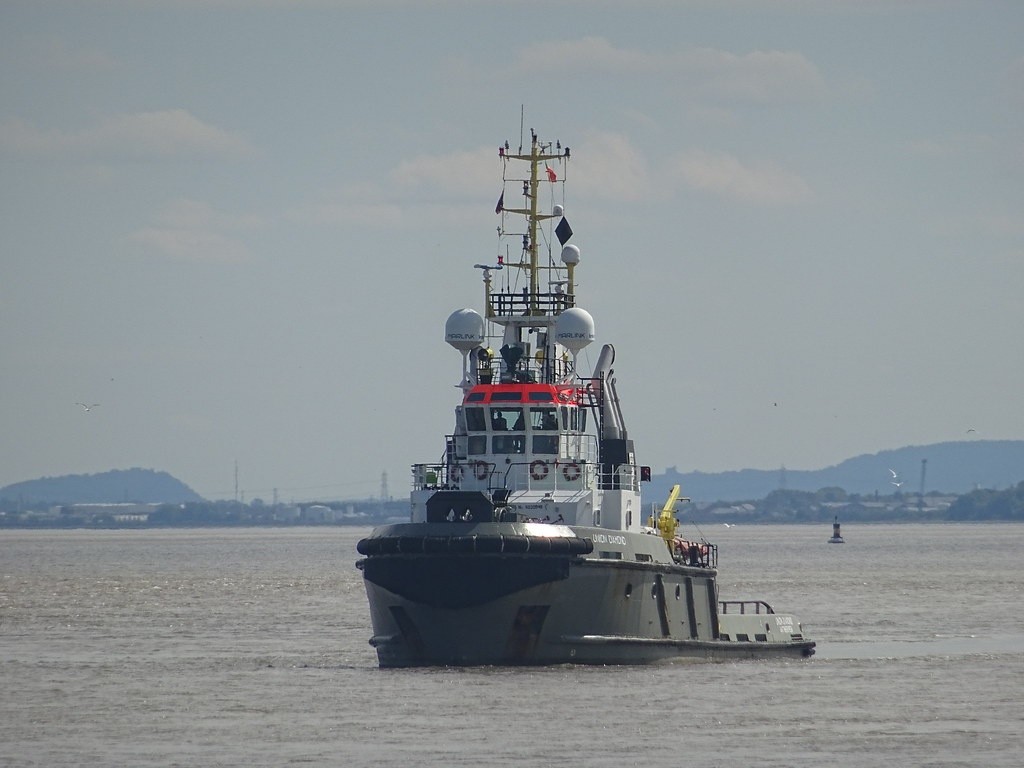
561;463;582;481
449;463;465;483
472;460;490;481
528;460;549;481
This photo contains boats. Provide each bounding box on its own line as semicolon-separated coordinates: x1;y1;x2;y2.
354;101;819;667
